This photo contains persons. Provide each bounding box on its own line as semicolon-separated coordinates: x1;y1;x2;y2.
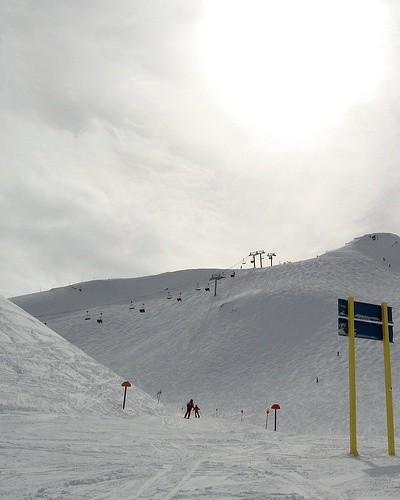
193;405;201;418
184;399;194;419
